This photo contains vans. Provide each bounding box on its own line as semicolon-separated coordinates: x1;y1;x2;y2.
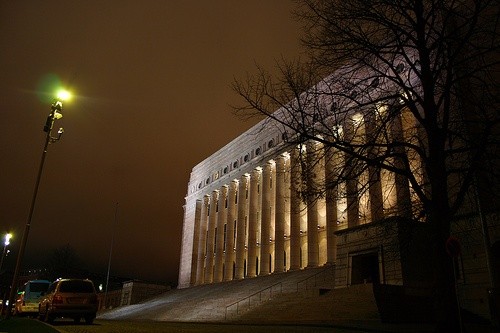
14;280;52;315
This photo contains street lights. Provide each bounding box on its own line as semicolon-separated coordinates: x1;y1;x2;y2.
4;89;72;318
1;233;12;270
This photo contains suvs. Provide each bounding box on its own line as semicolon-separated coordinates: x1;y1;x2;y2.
38;277;99;323
